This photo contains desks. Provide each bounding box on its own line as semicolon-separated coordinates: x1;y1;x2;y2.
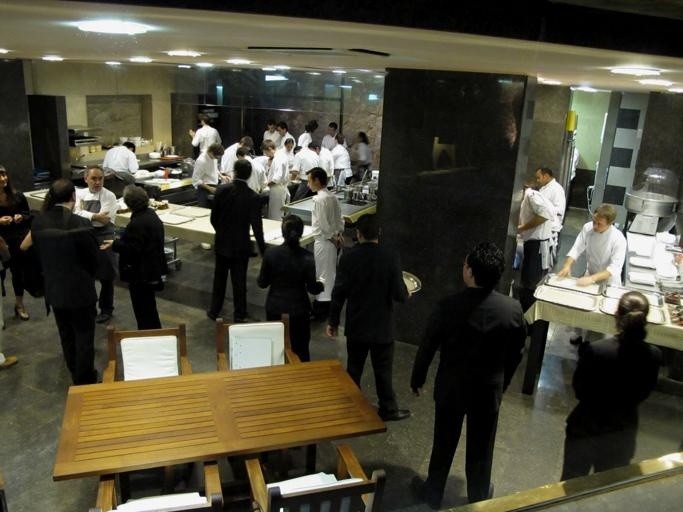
520;273;682;396
53;358;388;512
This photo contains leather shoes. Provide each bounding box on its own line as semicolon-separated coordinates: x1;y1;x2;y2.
486;483;493;499
380;410;409;421
411;476;423;498
95;313;109;323
2;356;17;369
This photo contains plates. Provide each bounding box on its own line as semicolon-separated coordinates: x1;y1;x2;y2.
629;256;656;270
629;271;656;286
399;269;423;294
652;251;677;281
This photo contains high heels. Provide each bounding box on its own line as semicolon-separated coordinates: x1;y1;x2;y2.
14;304;29;320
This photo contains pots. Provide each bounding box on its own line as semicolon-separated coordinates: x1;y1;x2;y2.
622;190;679;218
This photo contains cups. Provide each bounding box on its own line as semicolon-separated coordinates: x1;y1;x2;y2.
340;183;377;203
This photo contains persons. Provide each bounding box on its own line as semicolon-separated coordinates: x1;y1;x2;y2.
187;109;372;224
303;167;344;322
515;182;555;314
409;239;529;510
531;165;567;269
71;166;118;321
1;167;30;367
557;290;663;480
206;160;266;323
103;142;140;197
21;177;106;385
325;212;413;425
99;185;168;330
257;214;326;366
555;203;627;346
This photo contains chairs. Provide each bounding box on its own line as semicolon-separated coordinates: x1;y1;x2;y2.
243;443;386;512
100;321;192;384
216;313;301;373
89;458;224;512
